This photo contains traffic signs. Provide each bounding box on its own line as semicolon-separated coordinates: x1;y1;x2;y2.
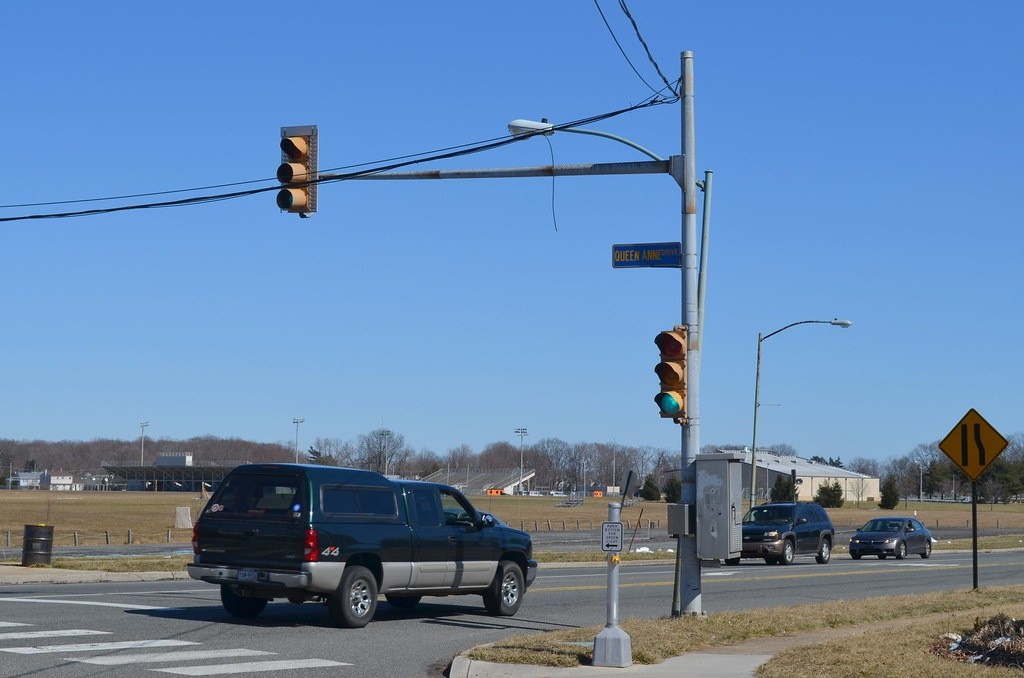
601;521;624;552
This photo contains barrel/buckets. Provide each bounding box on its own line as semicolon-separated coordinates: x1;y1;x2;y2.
21;525;54;568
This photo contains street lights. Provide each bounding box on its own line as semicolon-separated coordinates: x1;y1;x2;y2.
508;117;713;619
746;318;853;521
513;428;528;490
139;421;150;466
378;433;391;479
293;417;306;464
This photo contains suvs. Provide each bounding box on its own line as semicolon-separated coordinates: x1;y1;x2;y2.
184;462;539;628
722;500;835;567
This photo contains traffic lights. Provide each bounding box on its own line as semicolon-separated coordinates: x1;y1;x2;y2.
276;124;318;214
654;330;687;418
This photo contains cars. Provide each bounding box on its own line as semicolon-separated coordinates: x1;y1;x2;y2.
849;517;932;560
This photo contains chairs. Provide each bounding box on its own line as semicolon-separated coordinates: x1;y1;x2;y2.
880;525;889;530
898;526;907;533
